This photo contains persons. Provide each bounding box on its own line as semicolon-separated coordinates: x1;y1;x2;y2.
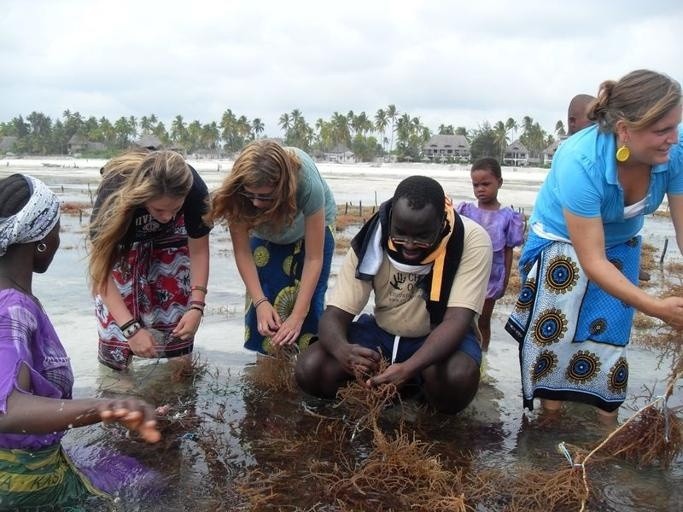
457;158;524;352
200;140;336;383
292;175;493;418
0;172;162;512
79;142;213;395
504;68;682;428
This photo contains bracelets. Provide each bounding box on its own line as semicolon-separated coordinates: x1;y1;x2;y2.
119;319;139;329
252;297;268;308
122;322;141;338
189;301;206;307
187;306;203;315
188;286;208;295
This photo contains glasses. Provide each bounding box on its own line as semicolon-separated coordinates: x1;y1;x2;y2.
238;191;275;202
388;221;442;250
